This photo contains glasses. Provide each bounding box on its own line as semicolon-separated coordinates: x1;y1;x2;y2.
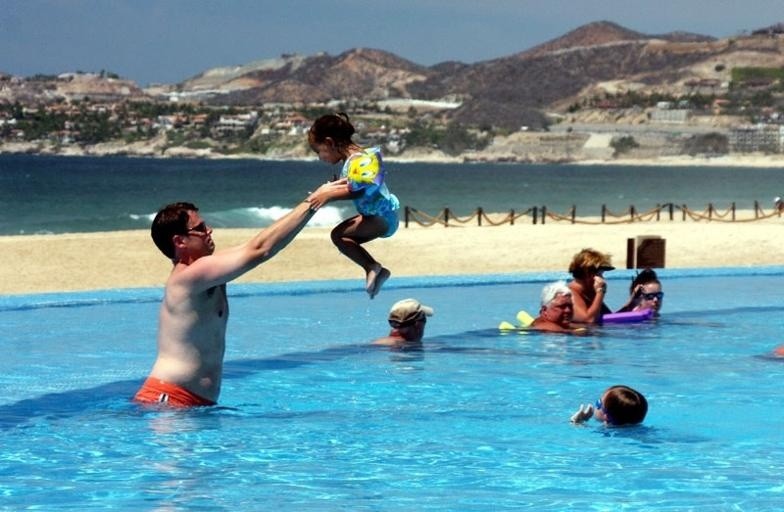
183;222;205;235
639;291;663;300
595;398;601;408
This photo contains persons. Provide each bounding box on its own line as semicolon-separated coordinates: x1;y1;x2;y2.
133;175;348;414
567;248;615;324
570;385;648;427
306;114;401;299
373;299;435;348
529;281;575;333
616;268;664;315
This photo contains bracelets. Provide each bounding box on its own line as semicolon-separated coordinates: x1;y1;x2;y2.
595;287;605;292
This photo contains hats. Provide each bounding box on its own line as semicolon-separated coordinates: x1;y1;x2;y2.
387;297;433;327
573;262;615;278
